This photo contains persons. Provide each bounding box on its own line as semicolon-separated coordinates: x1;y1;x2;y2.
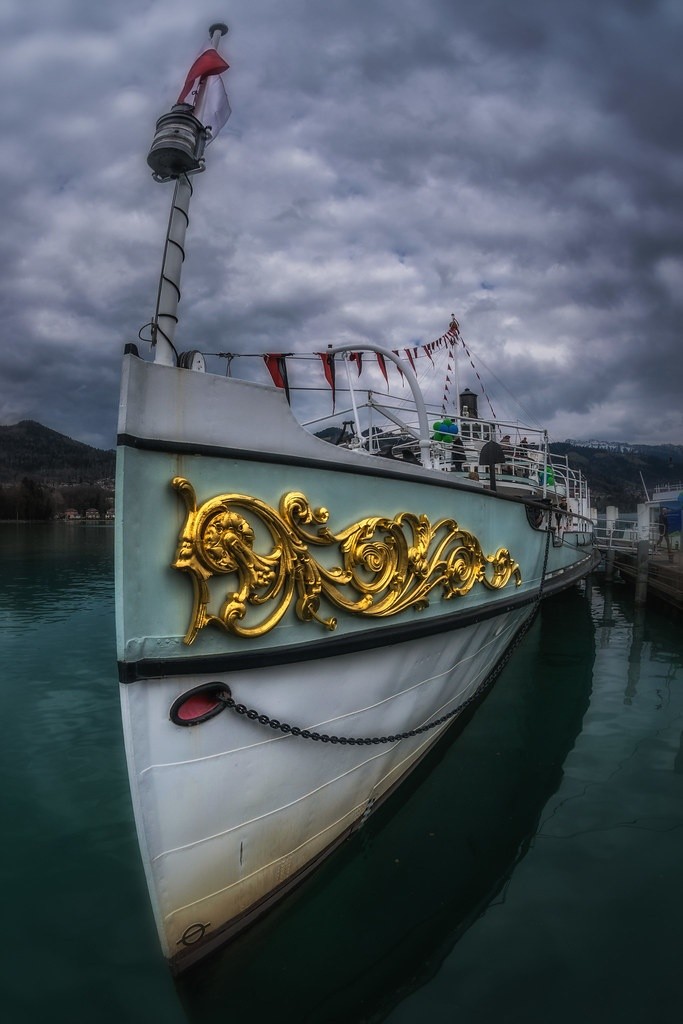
500;435;513;461
451;437;467;472
555;497;573;534
519;437;528;459
654;507;674;554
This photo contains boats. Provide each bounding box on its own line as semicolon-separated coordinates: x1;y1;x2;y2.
114;23;605;981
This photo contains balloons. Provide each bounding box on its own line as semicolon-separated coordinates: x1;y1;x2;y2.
539;467;555;486
432;418;458;444
